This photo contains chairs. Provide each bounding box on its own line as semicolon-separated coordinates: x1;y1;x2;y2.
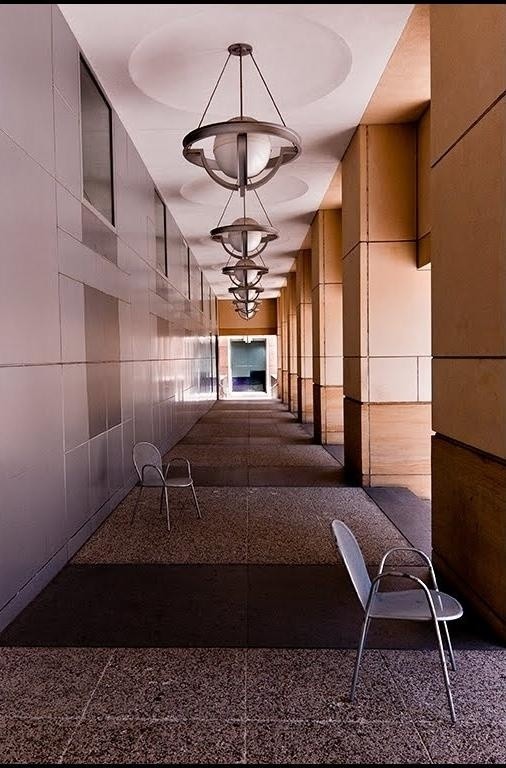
326;515;467;726
130;439;204;533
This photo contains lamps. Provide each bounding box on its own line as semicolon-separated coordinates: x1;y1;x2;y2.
178;42;306;201
206;173;278;260
220;245;269;321
